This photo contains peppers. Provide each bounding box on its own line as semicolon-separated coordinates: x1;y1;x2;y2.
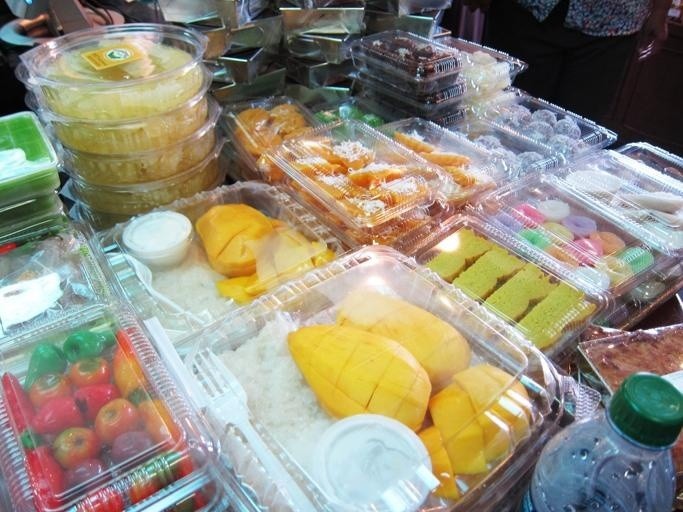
3;328;206;512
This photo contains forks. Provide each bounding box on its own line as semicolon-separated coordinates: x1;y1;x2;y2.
124;256;207;328
185;345;318;512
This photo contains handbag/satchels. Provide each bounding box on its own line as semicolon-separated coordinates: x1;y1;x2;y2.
488;0;558;57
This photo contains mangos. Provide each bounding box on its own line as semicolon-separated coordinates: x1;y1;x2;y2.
195;203;336;308
287;283;533;499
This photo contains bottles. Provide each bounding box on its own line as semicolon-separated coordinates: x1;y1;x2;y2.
516;373;683;511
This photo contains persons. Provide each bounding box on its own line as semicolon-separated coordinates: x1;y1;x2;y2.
462;0;673;120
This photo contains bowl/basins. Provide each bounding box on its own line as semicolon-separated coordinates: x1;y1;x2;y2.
123;209;192;270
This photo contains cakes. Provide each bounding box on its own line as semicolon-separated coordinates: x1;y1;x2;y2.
426;228;598;361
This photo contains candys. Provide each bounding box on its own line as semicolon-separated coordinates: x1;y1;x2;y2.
488;197;667;327
